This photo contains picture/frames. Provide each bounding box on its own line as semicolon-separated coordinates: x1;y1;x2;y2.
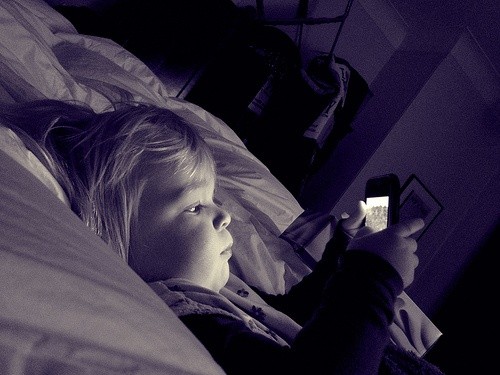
399;173;444;241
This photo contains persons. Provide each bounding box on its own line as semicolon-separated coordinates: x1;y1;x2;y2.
0;99;500;375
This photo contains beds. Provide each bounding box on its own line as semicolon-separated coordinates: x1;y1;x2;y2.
1;0;446;375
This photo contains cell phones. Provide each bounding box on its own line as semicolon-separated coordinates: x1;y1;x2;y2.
361;174;401;234
